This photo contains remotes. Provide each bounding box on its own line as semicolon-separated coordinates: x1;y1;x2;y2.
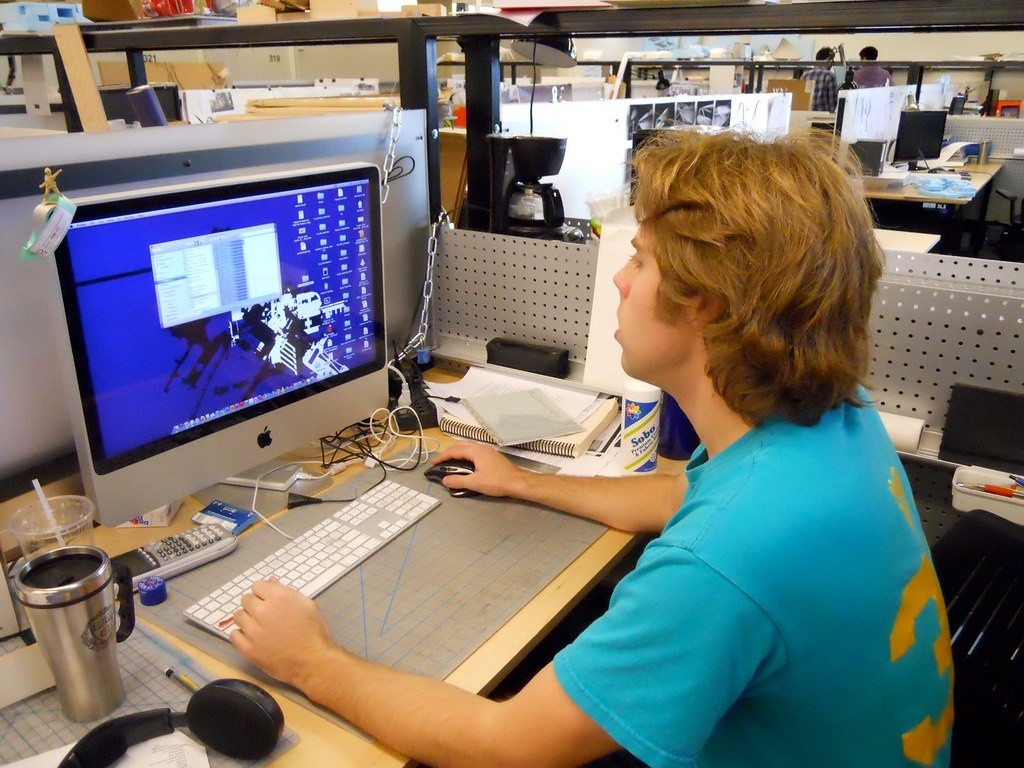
109;523;237;601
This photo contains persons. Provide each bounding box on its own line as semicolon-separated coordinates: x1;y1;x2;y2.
230;125;956;768
799;46;838;113
851;46;895;90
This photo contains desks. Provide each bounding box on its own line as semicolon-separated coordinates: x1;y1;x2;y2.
0;91;1004;768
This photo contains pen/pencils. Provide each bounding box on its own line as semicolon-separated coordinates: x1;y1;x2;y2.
956;482;1024;499
164;668;200;694
1009;475;1024;487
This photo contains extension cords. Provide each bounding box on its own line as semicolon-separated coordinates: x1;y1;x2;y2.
389;376;439;430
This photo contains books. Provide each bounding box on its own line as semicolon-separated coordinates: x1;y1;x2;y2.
440;388;619;459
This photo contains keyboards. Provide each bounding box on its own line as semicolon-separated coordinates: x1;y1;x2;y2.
182;481;441;641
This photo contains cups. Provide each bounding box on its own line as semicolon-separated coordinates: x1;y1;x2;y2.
622;378;660;475
14;546;135;721
8;495;95;563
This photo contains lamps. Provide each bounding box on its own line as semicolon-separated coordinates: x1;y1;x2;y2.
631;67;672;90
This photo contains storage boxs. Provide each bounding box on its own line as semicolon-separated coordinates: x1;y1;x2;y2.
75;4;93;23
0;2;52;32
48;3;76;24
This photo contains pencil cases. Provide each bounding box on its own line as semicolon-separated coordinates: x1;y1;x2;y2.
486;338;570;380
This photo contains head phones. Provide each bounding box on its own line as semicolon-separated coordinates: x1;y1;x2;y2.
57;678;284;768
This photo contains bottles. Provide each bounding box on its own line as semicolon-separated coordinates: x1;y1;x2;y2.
733;42;752;58
452;82;468;127
977;139;991;164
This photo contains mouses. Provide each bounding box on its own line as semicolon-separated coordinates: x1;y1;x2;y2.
423;457;478;497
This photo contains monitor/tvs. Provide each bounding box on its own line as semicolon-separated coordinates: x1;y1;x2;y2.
34;161;389;528
948;96;966;115
100;82;183;127
892;110;947;171
